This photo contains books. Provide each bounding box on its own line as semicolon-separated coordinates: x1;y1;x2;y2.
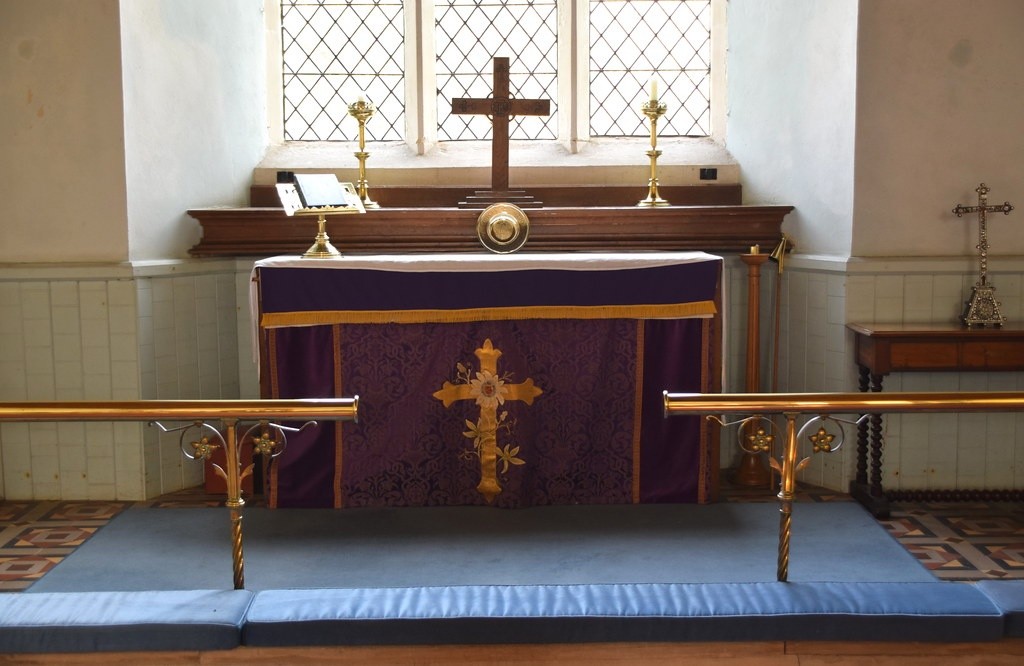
294;174;348;209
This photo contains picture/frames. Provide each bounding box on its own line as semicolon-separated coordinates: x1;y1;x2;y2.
275;182;366;216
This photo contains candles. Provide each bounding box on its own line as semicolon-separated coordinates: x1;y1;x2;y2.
358;91;365;101
750;244;760;254
651;79;657;100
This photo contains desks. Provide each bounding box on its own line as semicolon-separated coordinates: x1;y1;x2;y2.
846;322;1024;518
248;251;725;511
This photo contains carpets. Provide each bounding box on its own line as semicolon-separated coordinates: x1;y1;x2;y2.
22;500;942;593
